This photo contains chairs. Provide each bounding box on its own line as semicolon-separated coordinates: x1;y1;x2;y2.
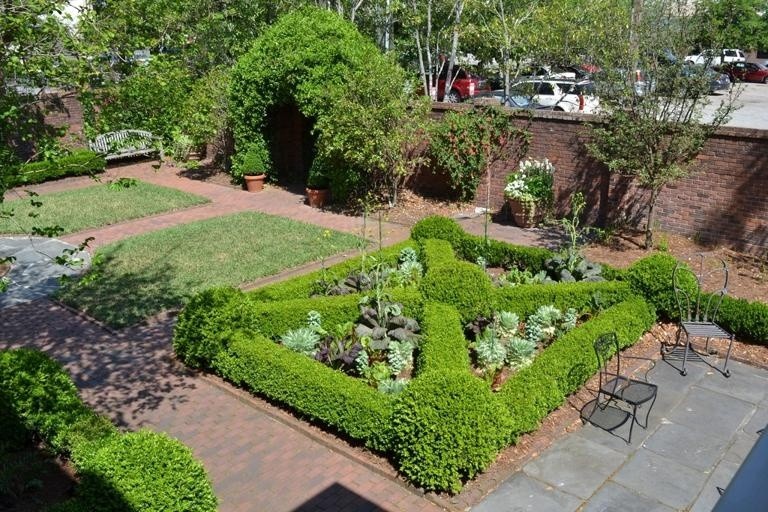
587;333;658;445
658;252;735;378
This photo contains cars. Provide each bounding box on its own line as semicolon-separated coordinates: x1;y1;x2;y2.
710;61;768;84
662;65;731;99
517;50;693;105
415;63;491;103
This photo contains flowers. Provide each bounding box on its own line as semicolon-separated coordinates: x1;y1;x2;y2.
503;157;559;225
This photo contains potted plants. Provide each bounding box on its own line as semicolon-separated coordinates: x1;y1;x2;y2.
305;157;329;209
241;142;268;192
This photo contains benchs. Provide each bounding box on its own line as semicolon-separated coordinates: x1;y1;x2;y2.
88;129;164;168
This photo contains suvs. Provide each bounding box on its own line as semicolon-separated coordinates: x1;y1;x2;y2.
682;48;746;69
485;78;600;115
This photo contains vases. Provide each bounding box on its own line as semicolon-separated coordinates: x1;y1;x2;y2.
507;196;548;228
